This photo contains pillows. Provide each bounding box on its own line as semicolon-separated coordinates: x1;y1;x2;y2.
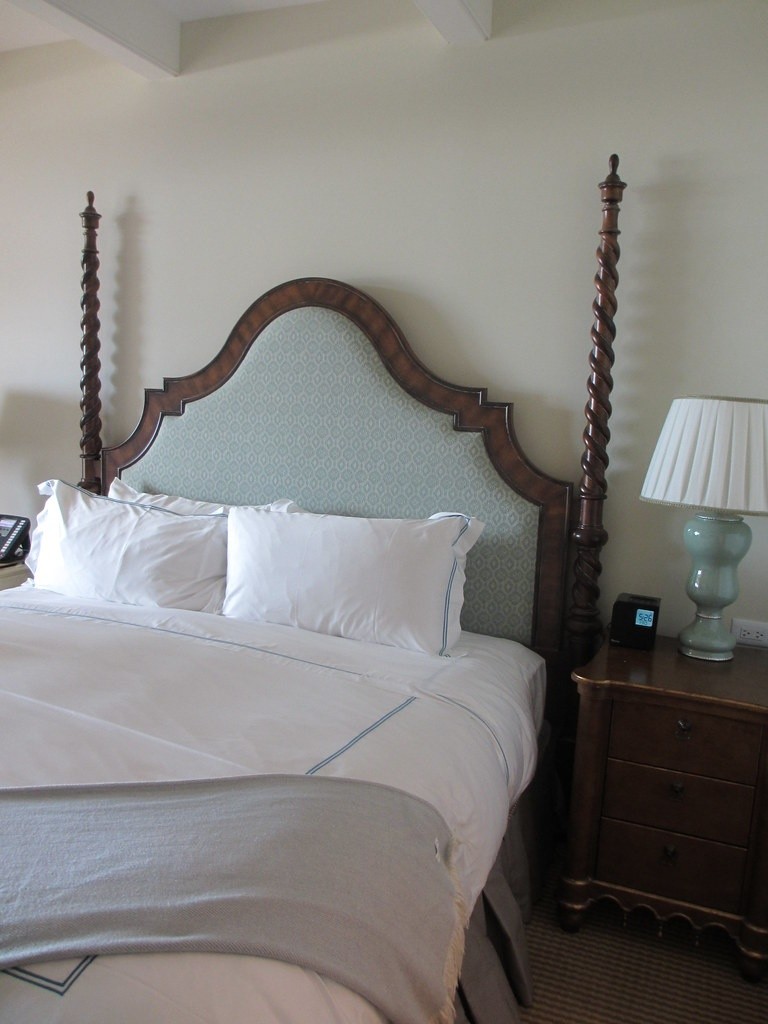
230;504;485;657
109;476;271;517
20;479;229;615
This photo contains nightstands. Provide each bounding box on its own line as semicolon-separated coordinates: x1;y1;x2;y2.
562;618;768;986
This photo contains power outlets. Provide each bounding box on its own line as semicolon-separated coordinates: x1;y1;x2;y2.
731;618;768;647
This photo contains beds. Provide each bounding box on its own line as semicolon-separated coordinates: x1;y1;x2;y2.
0;153;625;1024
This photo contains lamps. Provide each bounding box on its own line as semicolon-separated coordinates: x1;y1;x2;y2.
640;398;768;662
